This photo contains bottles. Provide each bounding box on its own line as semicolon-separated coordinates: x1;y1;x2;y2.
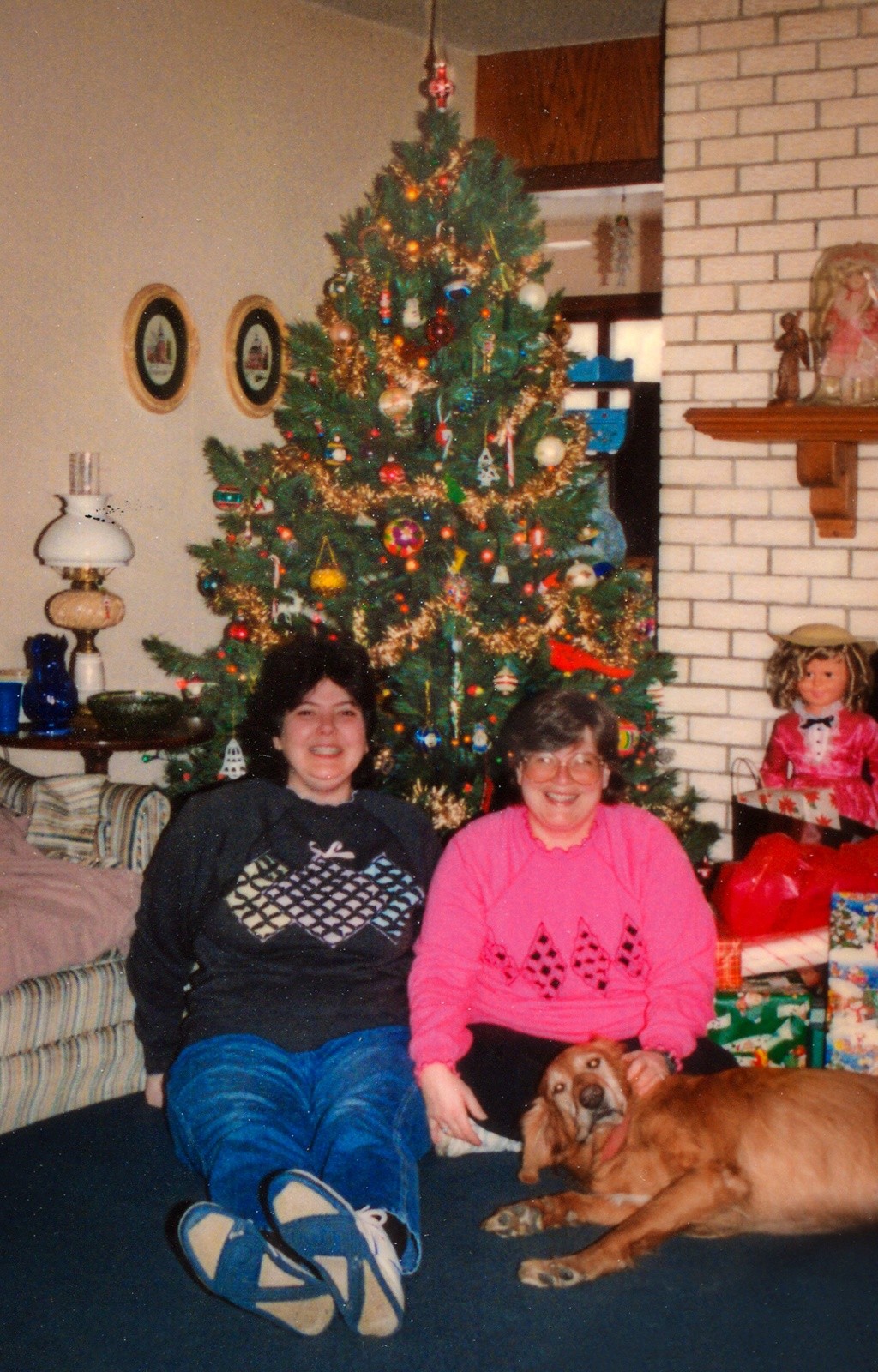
22;634;78;737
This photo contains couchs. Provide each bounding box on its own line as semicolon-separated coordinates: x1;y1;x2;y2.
0;763;170;1134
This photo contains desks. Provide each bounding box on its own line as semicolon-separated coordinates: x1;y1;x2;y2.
0;719;215;775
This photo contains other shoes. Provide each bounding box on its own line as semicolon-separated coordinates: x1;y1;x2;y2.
176;1203;337;1336
267;1167;408;1337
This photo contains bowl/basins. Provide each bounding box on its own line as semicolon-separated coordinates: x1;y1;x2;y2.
87;691;183;738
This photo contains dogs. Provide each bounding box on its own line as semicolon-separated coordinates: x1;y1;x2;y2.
485;1042;878;1291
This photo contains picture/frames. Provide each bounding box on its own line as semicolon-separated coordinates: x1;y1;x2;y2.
224;294;287;420
123;283;198;413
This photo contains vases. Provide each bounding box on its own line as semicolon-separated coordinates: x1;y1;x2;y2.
21;633;80;735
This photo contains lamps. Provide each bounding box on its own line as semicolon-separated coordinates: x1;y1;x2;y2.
35;451;134;716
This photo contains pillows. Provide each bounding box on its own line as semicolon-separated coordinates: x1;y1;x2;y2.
0;805;144;1001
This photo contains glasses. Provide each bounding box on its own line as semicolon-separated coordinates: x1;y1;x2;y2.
520;752;604;785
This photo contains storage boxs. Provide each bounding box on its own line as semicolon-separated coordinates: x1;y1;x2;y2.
708;978;813;1070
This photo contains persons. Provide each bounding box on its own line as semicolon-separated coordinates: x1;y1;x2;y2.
756;625;877;830
409;688;740;1159
775;313;810;405
816;262;878;405
123;635;456;1335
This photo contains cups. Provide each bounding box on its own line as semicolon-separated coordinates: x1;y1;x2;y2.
0;682;23;733
